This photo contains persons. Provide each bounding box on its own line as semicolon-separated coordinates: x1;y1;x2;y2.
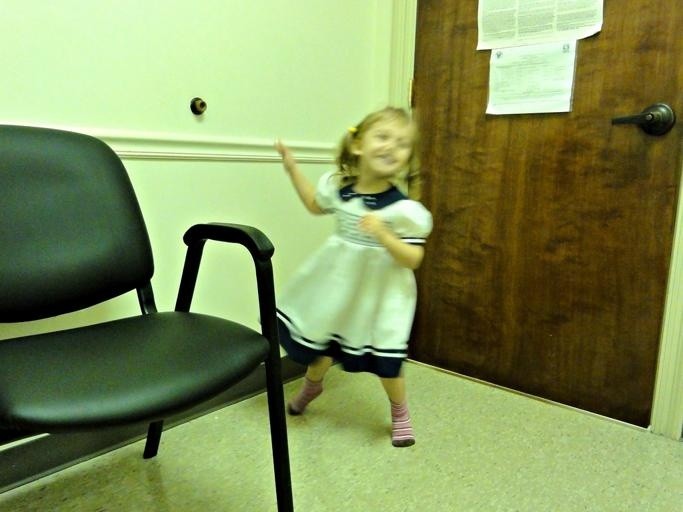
274;106;434;447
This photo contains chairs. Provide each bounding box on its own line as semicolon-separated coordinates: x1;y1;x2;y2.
0;123;295;512
